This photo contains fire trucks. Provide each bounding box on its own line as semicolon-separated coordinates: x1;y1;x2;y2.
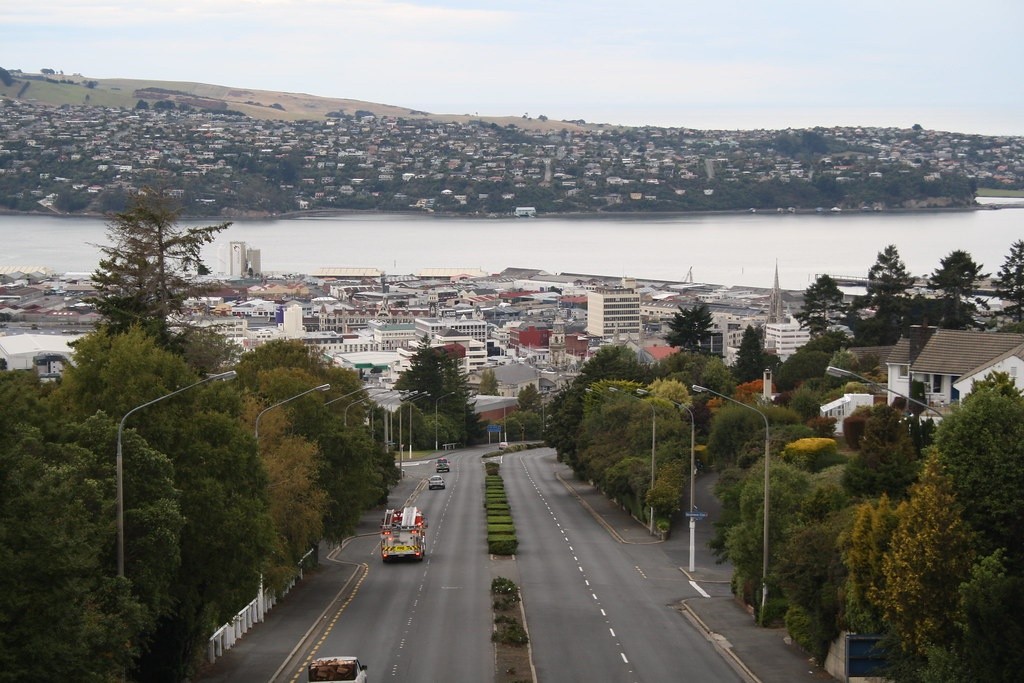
381;507;428;562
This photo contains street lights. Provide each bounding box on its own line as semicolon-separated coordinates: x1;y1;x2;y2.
371;390;409;438
344;388;389;427
399;391;428;468
692;384;770;606
117;369;238;581
609;386;656;536
255;383;331;442
637;388;695;573
409;394;431;458
435;392;456;451
384;390;417;454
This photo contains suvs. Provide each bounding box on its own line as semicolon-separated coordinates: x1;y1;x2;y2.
435;458;450;473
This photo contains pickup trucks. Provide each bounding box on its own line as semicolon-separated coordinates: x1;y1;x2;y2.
308;655;368;683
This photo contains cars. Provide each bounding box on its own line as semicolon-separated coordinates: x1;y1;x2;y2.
499;441;510;450
428;476;446;490
